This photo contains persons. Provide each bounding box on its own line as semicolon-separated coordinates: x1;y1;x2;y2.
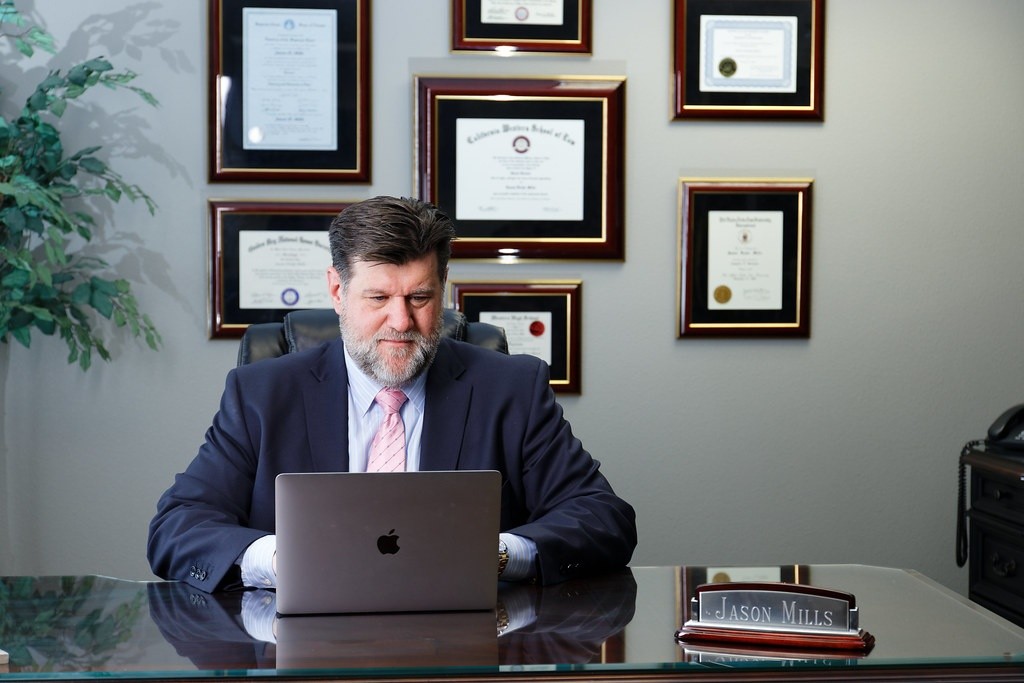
146;194;638;593
145;568;637;665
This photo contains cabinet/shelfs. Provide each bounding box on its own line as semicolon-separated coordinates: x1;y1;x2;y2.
962;449;1024;628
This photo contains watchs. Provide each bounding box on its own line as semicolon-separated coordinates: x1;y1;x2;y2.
495;599;509;635
498;539;509;572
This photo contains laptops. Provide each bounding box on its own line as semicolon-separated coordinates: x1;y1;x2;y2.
274;469;503;617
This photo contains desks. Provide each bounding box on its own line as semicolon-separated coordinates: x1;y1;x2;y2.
0;563;1024;682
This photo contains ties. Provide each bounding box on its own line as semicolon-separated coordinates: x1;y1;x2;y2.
366;386;407;471
